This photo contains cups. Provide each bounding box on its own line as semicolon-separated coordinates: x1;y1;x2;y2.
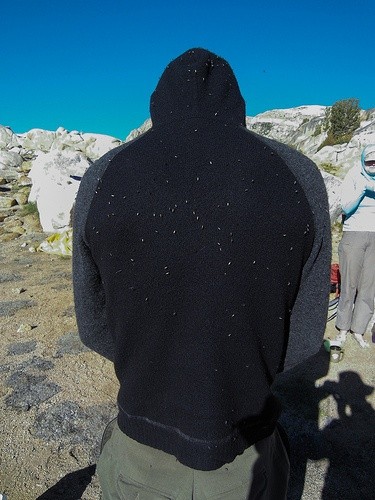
329;345;344;363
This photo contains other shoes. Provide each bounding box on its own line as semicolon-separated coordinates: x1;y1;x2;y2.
352;332;370;348
337;330;349;347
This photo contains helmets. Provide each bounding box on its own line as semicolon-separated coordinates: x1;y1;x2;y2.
361;144;375;176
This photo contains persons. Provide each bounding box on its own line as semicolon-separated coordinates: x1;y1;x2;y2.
67;48;335;500
327;141;375;350
